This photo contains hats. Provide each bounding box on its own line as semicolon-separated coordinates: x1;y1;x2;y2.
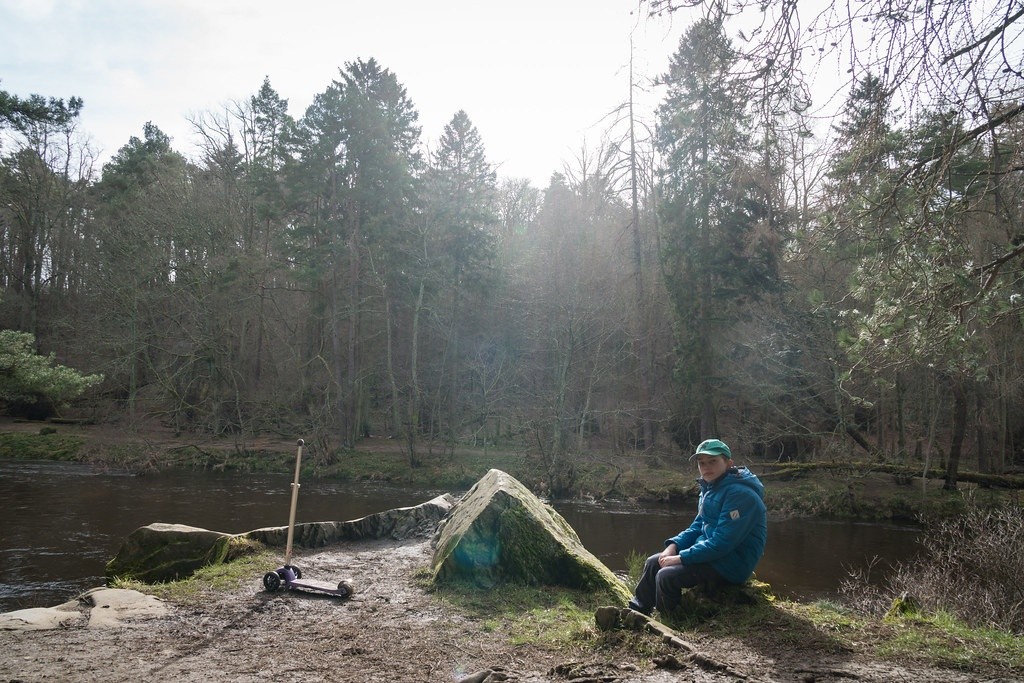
690;439;731;462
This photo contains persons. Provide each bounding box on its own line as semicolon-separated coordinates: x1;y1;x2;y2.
628;439;767;624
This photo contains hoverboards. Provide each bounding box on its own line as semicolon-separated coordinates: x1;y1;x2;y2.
262;442;354;599
